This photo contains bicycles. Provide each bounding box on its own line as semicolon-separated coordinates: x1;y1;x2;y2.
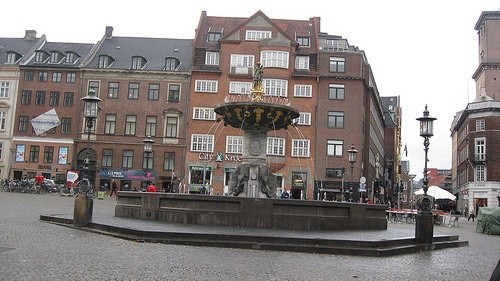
0;177;98;200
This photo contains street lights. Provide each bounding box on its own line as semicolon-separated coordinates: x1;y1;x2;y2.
74;85;103;224
413;104;437;245
346;144;358;202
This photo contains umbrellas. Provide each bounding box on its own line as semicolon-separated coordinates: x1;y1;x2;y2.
414;184;456;212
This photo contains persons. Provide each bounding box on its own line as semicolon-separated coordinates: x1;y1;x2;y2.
333;193;405;219
281;188;289;199
136;179;211;195
109;178;119;198
468;205;475;221
